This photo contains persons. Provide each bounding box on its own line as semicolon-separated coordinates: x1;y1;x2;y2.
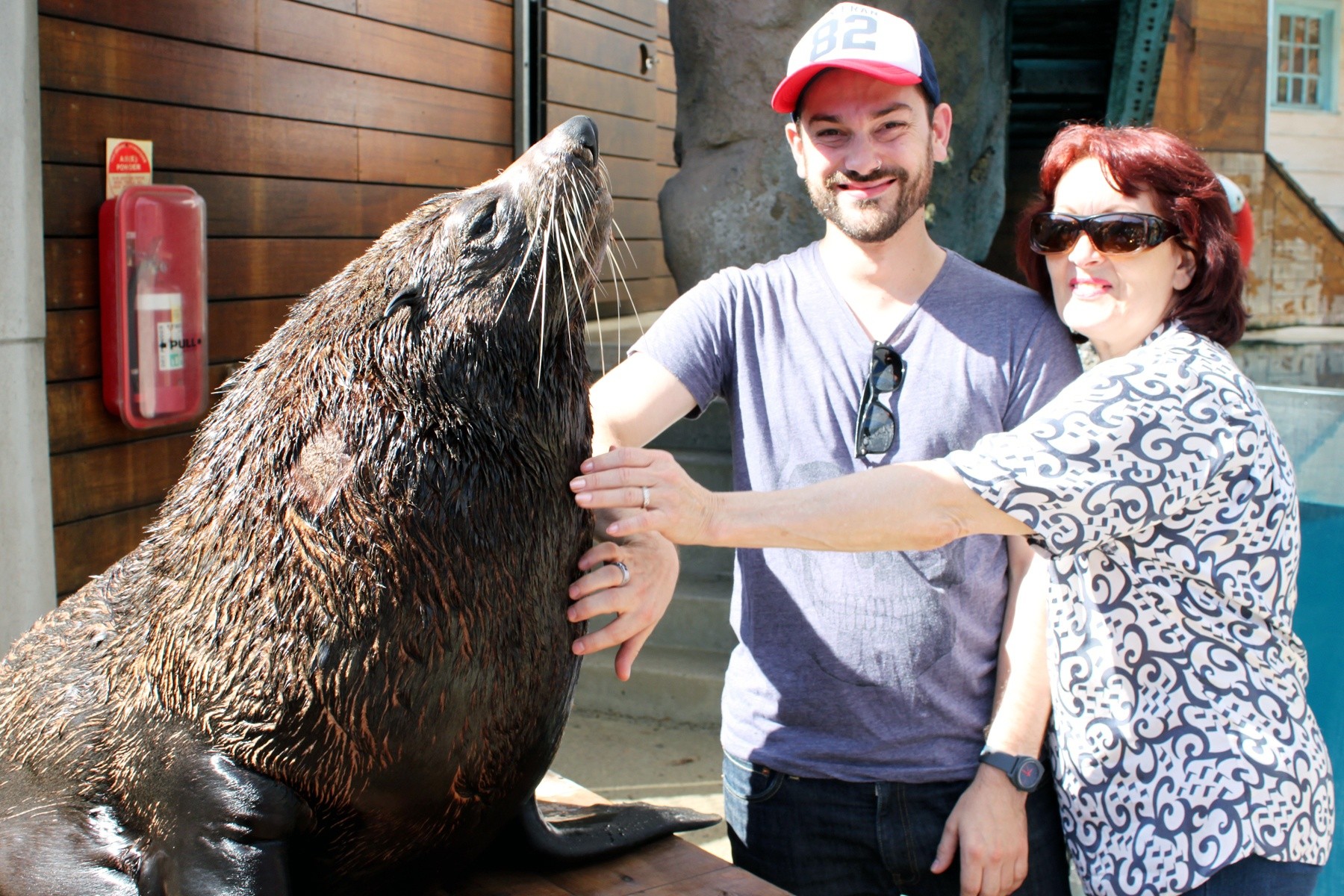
570;123;1334;896
567;1;1088;896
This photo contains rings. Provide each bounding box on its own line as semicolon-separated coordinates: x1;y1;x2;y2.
608;561;632;587
640;488;650;508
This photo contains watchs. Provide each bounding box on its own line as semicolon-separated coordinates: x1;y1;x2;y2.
977;743;1044;791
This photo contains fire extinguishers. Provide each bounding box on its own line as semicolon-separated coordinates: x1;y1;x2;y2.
122;235;190;414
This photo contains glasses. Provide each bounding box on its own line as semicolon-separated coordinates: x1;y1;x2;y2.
853;340;904;459
1026;209;1181;257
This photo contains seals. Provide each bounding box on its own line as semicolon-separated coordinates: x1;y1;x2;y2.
2;111;721;895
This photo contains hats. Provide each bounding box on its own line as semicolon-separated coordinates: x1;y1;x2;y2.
770;2;940;112
